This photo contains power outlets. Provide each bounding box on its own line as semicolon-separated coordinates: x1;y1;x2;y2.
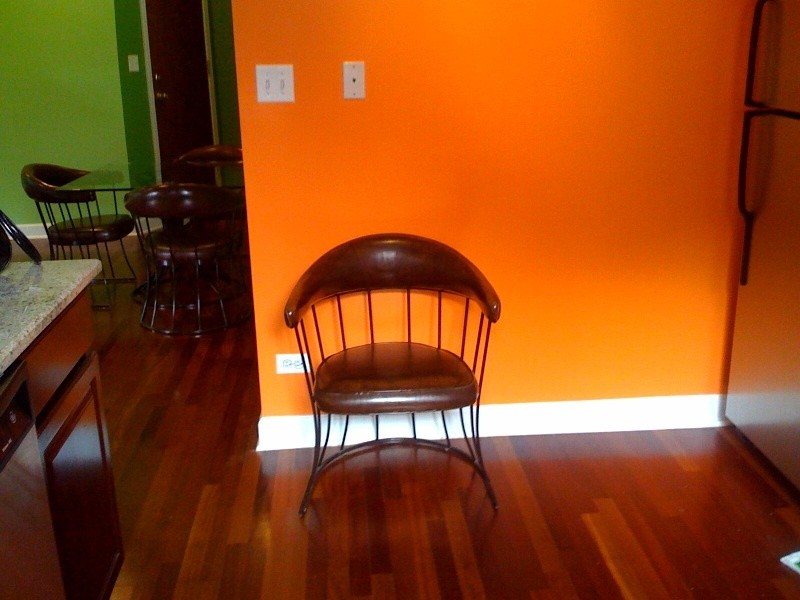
276;354;311;374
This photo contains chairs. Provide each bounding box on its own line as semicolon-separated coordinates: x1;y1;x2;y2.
21;144;253;337
284;232;501;519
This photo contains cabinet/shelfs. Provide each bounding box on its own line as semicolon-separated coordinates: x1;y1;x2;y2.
0;288;125;600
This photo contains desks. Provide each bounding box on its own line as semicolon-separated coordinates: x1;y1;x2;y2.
57;168;246;281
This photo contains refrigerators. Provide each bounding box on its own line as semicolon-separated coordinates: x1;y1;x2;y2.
723;0;800;489
